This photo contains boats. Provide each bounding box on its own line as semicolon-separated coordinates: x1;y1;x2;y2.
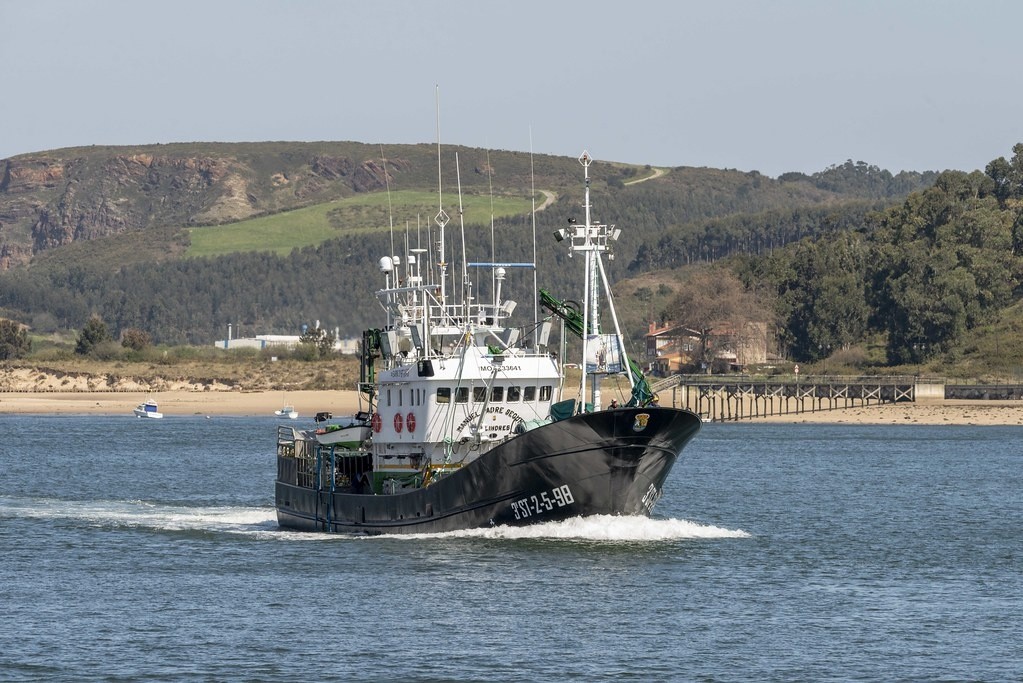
275;405;299;419
134;400;162;419
275;78;703;538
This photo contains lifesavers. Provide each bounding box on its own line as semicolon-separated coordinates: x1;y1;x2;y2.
595;349;607;372
406;412;415;433
371;413;382;433
393;412;403;433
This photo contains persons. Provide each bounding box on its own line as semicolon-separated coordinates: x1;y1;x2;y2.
607;399;622;410
645;392;661;408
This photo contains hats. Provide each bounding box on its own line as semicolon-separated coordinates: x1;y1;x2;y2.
650;395;659;401
611;398;617;402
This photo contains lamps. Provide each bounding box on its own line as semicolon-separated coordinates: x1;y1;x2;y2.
552;228;570;242
607;228;621;241
